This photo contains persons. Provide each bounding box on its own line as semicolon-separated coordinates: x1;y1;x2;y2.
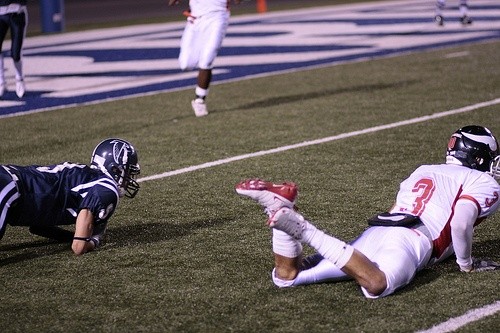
0;138;140;256
235;125;500;299
167;0;231;117
0;0;29;98
434;0;472;26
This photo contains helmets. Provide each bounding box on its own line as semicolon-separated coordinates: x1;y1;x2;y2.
445;125;500;172
91;137;139;198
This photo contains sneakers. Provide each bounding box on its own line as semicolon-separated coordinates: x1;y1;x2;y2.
15;79;26;99
459;16;472;25
190;98;208;118
433;14;444;27
234;178;298;214
0;78;7;97
268;206;307;240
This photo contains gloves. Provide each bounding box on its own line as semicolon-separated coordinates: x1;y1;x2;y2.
90;229;108;248
460;257;500;273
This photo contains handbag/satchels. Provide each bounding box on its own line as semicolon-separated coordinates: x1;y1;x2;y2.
367;211;422;228
